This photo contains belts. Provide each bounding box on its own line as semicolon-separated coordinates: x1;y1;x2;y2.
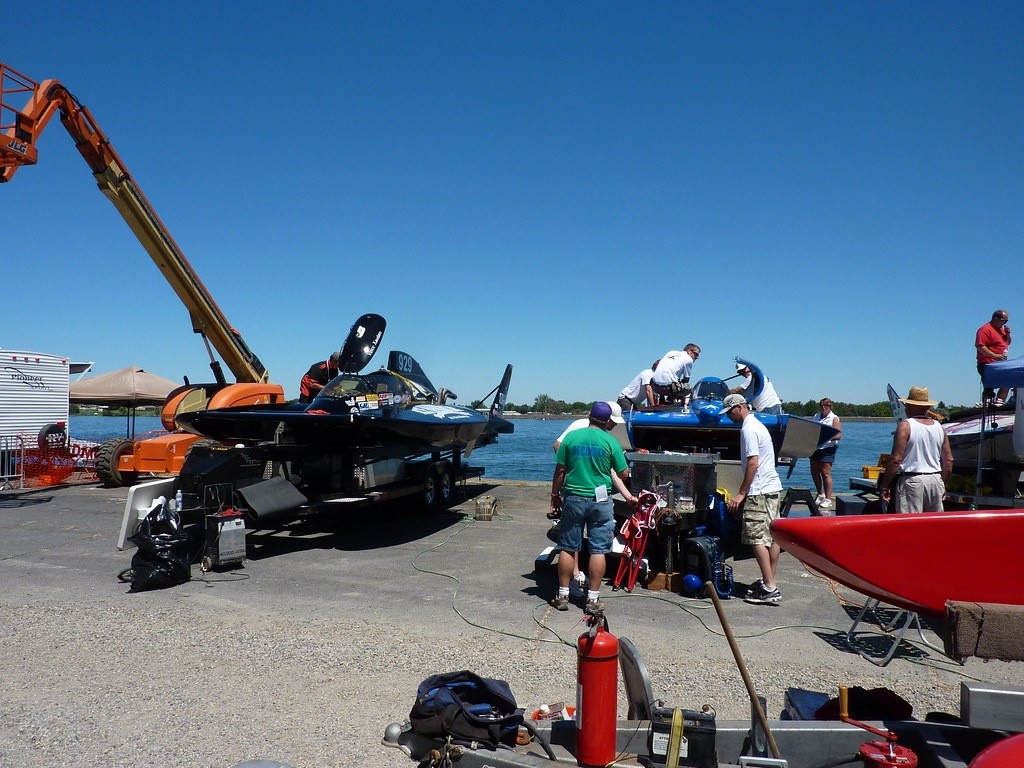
900;471;942;476
650;378;672;388
624;395;635;406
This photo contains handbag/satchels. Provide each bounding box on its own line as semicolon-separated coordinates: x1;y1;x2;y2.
409;668;524;746
124;517;191;594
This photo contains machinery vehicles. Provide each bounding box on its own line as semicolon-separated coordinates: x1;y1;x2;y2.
1;59;286;488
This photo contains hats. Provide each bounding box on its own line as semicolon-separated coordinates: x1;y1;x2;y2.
607;401;626;424
591;402;612;421
899;386;937;406
717;394;746;415
735;362;747;373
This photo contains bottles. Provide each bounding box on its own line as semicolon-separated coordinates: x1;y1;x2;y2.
539;702;568;720
176;490;183;511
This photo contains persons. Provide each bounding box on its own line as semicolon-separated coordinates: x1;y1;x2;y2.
881;385;954;513
548;402;631;612
975;310;1011;403
616;359;661;411
650;343;701;406
807;398;842;507
298;352;342;403
728;357;782;414
718;394;784;602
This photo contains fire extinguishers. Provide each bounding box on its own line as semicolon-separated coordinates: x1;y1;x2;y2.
575;597;620;768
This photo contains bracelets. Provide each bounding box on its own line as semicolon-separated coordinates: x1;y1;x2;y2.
551;494;559;496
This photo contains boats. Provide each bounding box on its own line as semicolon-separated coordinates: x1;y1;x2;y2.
617;377;840;460
887;382;1024;464
172;351;515;445
767;508;1024;618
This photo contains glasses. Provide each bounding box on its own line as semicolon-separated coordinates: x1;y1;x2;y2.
690;349;700;360
998;318;1008;323
819;405;831;408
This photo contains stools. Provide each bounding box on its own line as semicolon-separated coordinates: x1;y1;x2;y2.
779;486;820;519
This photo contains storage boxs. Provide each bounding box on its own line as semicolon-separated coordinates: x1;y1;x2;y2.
862;465;883;479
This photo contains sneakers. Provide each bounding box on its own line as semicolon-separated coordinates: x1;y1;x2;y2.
548;592;570;611
819;498;832;508
745;578;763;593
743;585;782;604
814;493;825;505
584;597;606;611
572;570;590;585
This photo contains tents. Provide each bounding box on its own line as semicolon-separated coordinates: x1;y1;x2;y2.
972;357;1024;510
68;366;183;440
